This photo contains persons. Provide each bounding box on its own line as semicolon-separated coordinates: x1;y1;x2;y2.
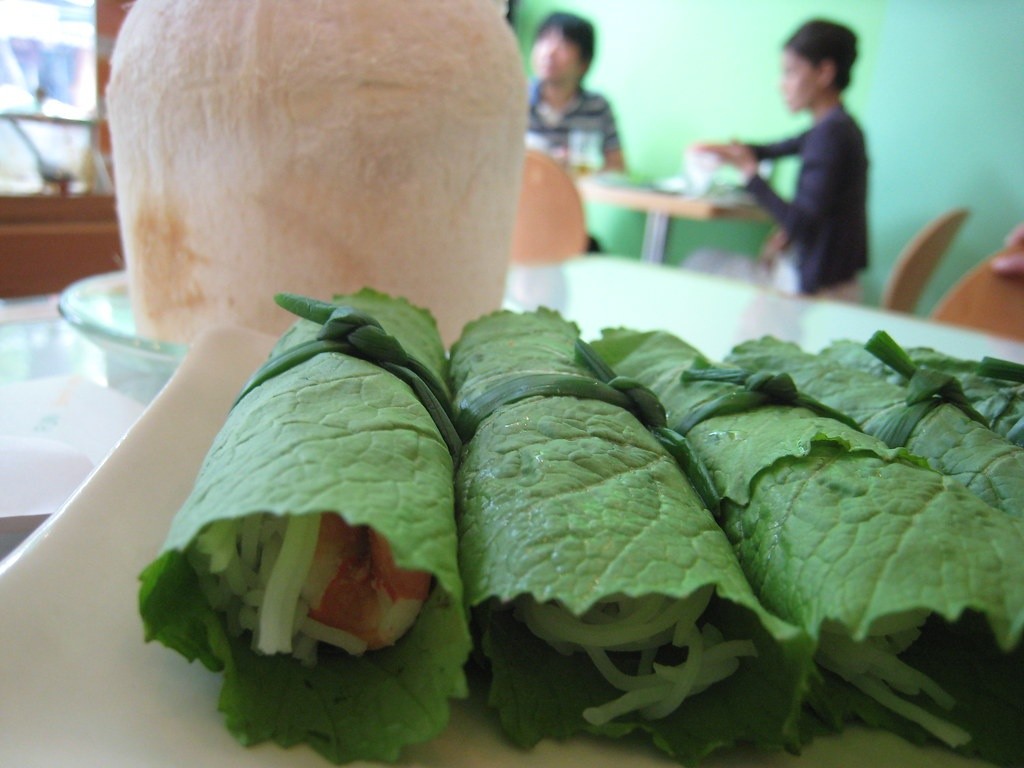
679;18;870;304
522;13;626;255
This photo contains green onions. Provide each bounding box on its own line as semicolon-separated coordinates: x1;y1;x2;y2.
862;330;989;449
451;335;722;516
978;356;1024;450
670;369;865;438
228;291;462;467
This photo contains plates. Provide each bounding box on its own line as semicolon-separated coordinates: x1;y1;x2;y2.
0;323;1024;768
56;265;184;374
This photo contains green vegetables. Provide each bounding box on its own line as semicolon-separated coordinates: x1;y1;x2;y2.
134;286;1024;767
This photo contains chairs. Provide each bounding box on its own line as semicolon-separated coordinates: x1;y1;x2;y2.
508;152;588;264
928;245;1024;343
798;209;971;313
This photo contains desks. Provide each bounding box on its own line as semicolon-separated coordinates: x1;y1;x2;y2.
0;256;1024;554
575;182;771;264
